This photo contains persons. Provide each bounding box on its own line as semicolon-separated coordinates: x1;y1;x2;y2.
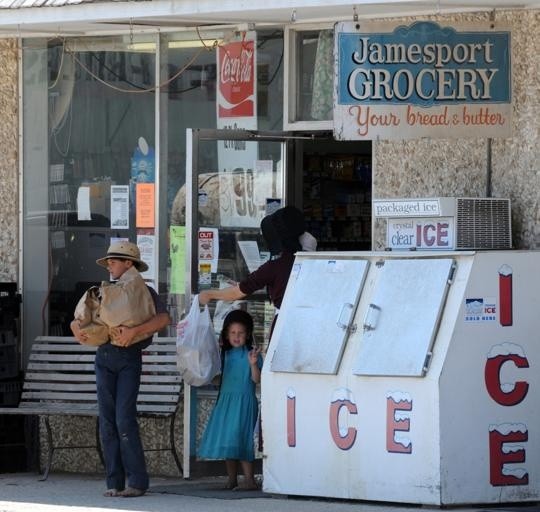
197;309;264;494
69;241;171;498
197;206;310;358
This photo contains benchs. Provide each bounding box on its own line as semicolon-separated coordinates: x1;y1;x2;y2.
0;336;184;482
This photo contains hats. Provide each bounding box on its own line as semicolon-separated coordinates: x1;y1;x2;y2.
96;241;149;272
222;310;254;343
